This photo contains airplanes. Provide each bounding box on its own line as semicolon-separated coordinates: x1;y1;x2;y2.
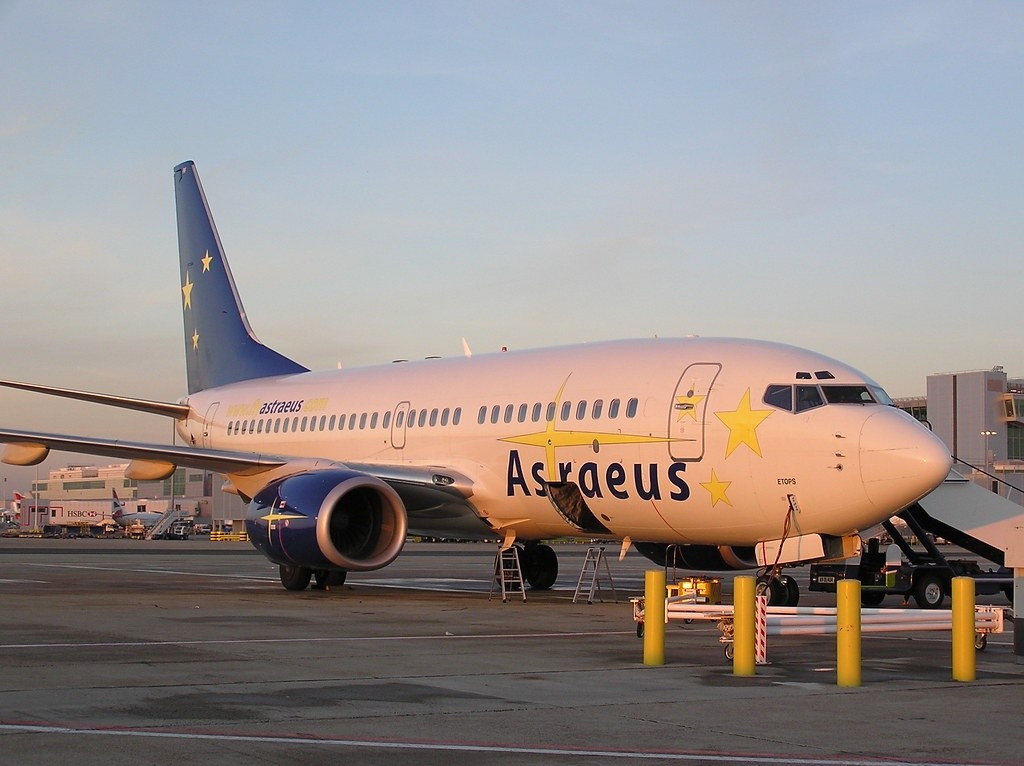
1;160;1024;607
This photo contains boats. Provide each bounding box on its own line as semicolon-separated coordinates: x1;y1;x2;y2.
112;488;189;541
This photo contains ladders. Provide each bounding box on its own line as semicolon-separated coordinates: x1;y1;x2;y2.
572;546;619;605
488;546;527;603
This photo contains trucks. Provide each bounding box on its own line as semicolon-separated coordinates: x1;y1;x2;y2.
172;526;190;540
195;523;211;535
